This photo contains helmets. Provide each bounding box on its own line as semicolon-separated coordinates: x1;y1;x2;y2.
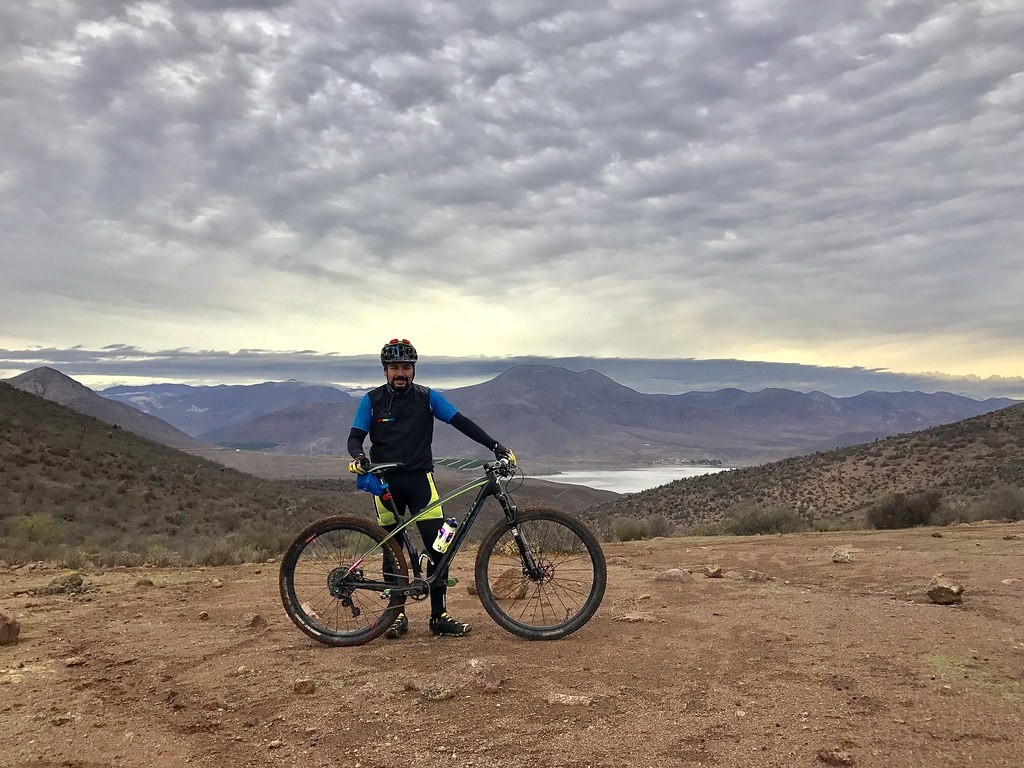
381;339;418;367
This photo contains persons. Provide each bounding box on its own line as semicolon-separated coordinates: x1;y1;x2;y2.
347;339;516;639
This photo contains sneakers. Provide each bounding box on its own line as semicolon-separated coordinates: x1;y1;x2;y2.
429;612;472;637
386;613;408;639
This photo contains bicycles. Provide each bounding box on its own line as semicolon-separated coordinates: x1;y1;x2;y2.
278;454;607;648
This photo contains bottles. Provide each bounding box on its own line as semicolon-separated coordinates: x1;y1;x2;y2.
432;517;458;554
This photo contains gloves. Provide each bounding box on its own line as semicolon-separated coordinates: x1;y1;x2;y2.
348;454;370;475
494;443;517;465
